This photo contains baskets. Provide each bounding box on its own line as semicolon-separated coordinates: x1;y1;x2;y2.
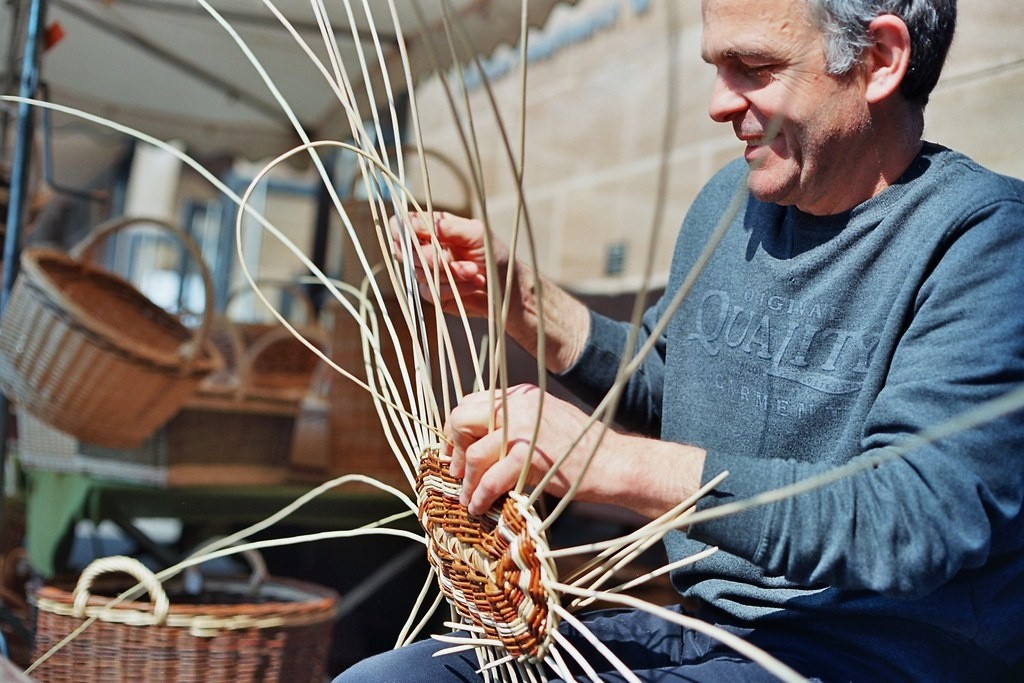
1;215;225;451
30;535;340;683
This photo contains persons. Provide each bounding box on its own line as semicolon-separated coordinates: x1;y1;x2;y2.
329;0;1024;682
